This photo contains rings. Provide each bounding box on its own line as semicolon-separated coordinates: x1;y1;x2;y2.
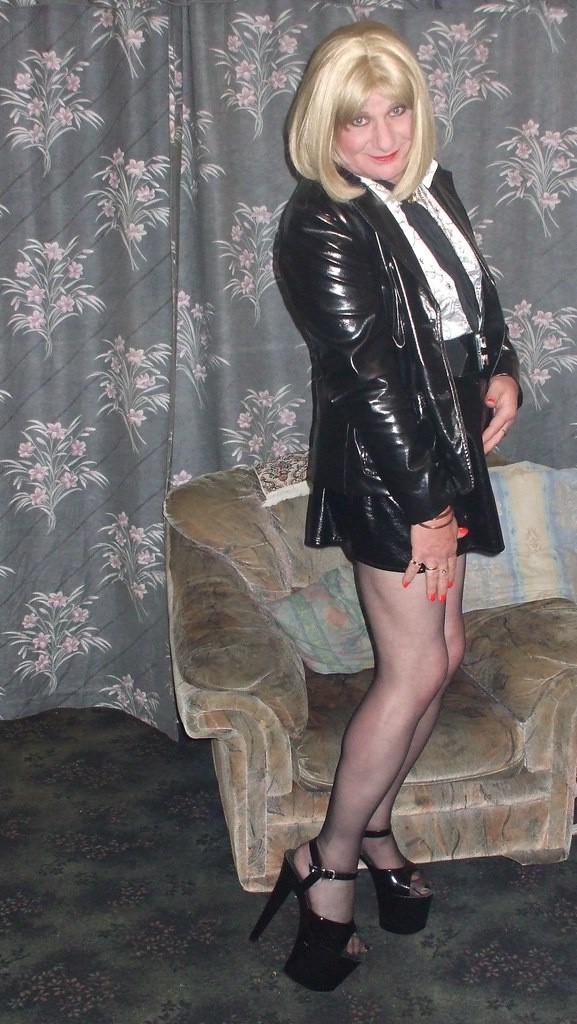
440;569;447;573
501;428;507;437
411;560;421;565
425;568;437;570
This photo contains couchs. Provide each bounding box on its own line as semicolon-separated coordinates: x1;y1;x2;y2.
163;445;577;893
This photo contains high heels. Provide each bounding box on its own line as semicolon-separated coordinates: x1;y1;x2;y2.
250;837;370;994
357;826;432;935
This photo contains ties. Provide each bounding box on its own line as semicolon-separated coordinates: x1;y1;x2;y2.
372;180;480;333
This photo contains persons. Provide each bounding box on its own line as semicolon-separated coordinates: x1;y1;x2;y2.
250;22;523;991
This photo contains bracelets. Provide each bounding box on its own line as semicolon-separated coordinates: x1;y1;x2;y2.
419;508;453;529
